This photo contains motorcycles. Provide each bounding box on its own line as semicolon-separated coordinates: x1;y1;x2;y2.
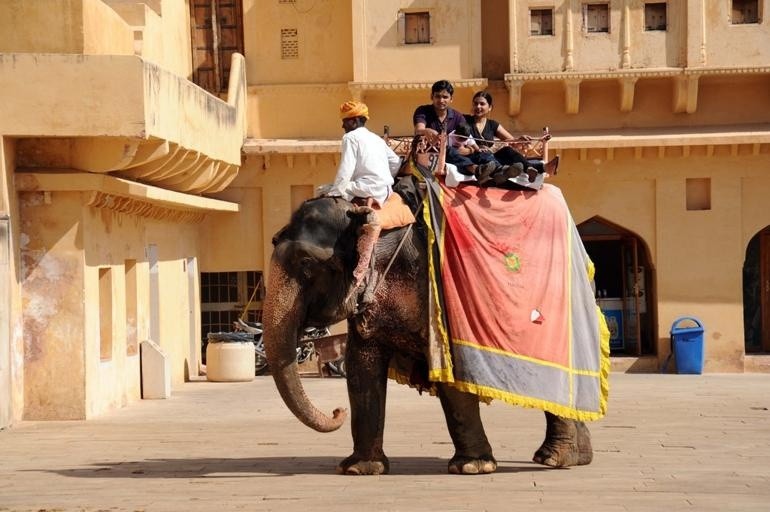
232;318;331;374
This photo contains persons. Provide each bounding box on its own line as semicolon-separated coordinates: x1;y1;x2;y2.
463;91;559;184
412;80;524;186
314;99;403;205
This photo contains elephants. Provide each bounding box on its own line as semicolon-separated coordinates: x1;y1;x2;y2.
261;183;593;475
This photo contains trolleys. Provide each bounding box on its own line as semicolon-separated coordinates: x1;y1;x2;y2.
303;333;348;378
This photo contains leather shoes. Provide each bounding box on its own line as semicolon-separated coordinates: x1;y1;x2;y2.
475;156;559;184
